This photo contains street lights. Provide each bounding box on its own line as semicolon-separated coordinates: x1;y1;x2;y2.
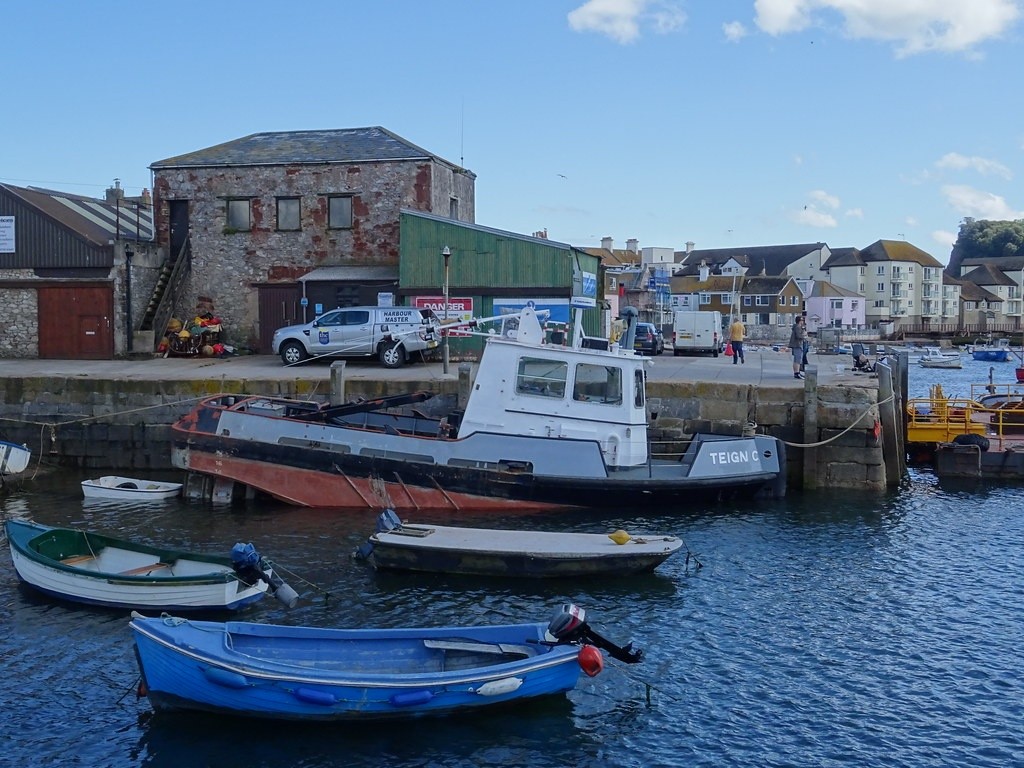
441;247;451;372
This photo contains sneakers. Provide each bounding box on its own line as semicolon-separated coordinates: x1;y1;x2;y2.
794;372;805;380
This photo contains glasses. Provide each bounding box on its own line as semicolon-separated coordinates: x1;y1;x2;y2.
800;319;805;321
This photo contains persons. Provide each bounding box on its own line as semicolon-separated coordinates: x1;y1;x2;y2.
792;325;808;377
724;318;745;364
788;316;805;379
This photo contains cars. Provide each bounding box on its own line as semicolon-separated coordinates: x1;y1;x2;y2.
633;323;665;355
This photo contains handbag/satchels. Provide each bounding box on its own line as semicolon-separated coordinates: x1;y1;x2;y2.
724;341;734;355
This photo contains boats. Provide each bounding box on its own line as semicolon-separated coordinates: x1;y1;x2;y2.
972;344;1010;362
3;516;299;615
918;349;962;368
81;475;184;498
980;367;1023;406
172;307;780;507
351;509;684;577
127;605;643;721
833;342;973;363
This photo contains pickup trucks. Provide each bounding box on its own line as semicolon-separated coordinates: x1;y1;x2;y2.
272;305;442;368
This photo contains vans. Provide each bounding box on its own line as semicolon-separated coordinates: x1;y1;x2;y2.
673;310;724;357
